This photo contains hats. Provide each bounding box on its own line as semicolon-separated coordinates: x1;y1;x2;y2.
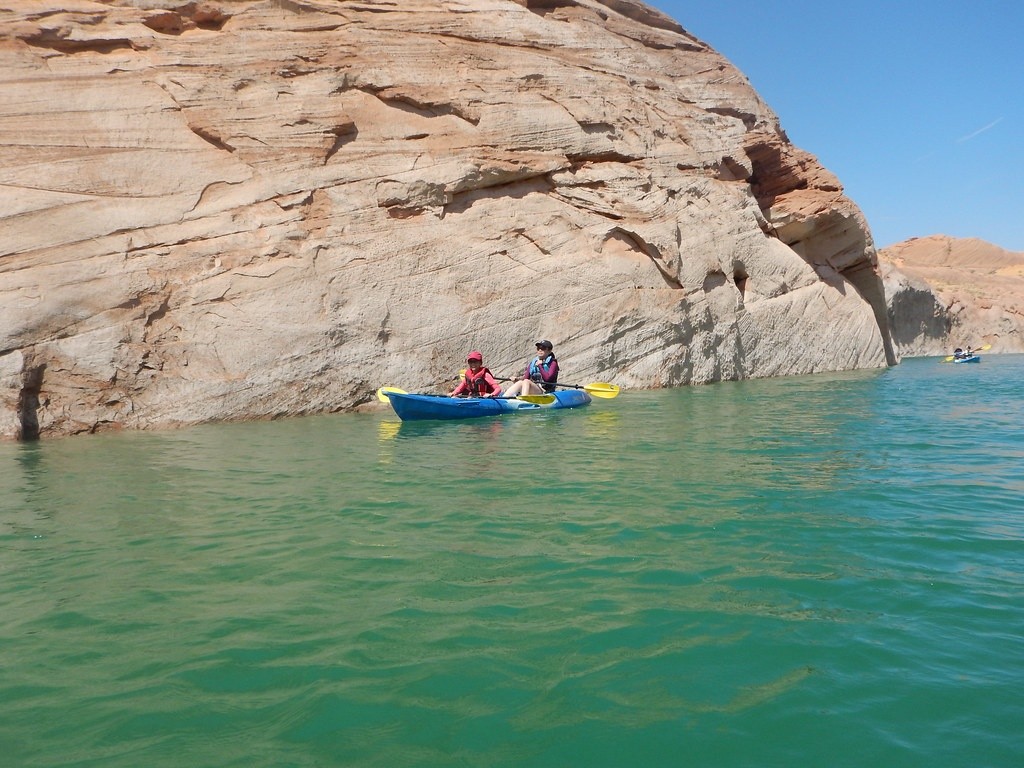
467;351;482;361
535;340;553;348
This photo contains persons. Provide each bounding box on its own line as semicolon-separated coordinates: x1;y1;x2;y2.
963;346;975;359
448;351;501;399
502;340;559;397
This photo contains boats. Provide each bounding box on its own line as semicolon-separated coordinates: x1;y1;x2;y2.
953;354;981;365
382;388;592;421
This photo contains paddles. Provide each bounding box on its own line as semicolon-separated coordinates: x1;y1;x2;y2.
459;368;619;399
946;343;992;362
377;384;555;405
